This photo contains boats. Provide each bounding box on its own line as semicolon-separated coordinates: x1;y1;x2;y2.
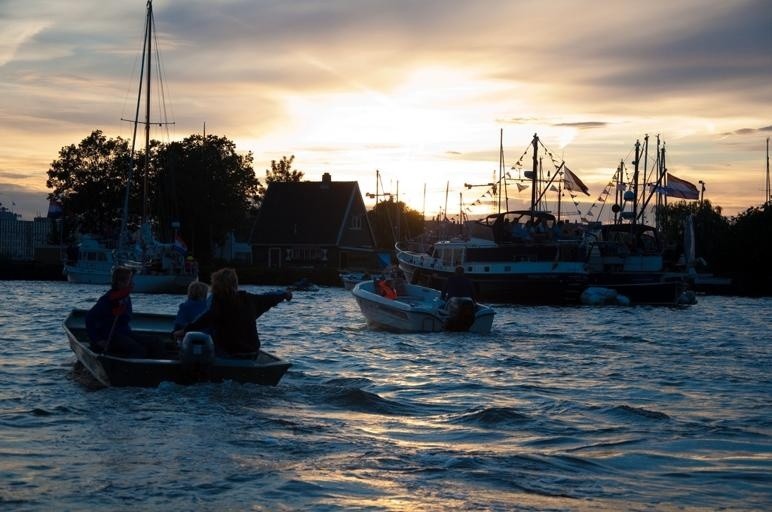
62;308;294;387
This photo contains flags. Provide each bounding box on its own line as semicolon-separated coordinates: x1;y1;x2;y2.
517;165;699;201
175;235;188;256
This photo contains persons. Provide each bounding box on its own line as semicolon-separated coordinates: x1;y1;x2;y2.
504;218;587;262
165;251;200;273
360;263;407;302
85;267;149;360
609;231;658;272
438;264;478;317
171;266;293;363
170;282;216;360
65;243;80;267
